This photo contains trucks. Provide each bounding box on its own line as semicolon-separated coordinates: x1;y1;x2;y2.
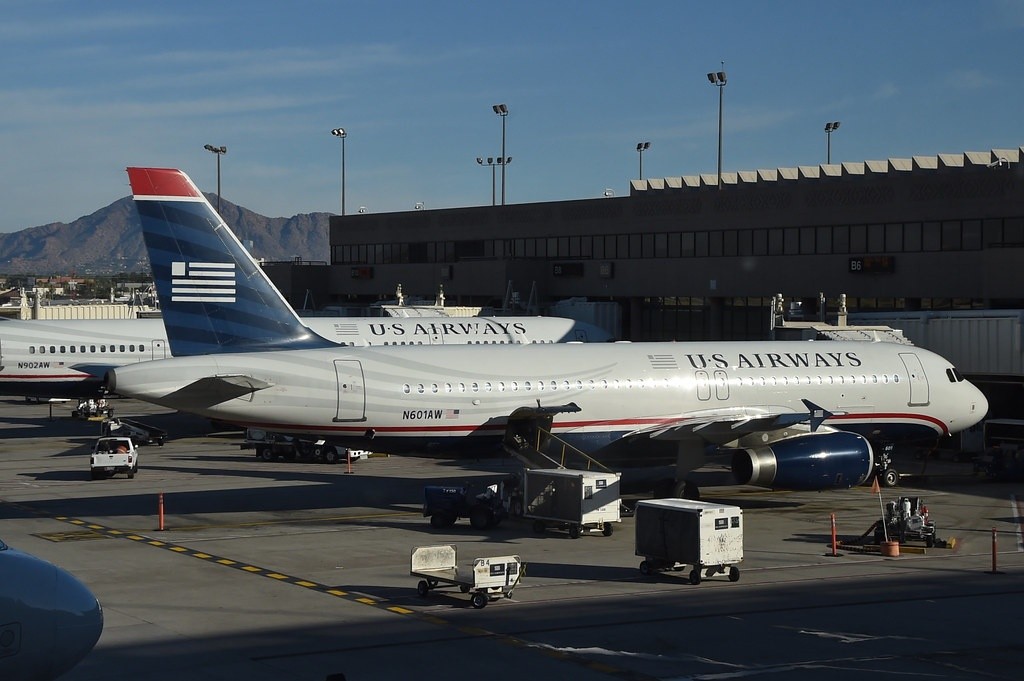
422;468;621;538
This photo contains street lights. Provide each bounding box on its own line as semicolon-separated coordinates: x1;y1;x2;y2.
331;128;347;216
825;122;842;164
493;104;509;206
637;142;651;180
204;144;227;215
707;61;727;190
476;157;512;206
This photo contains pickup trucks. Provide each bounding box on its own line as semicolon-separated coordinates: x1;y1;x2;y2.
89;437;139;479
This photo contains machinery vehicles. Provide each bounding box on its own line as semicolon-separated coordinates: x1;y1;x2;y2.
875;496;935;548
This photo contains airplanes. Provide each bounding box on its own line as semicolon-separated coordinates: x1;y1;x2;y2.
1;317;617;404
104;168;989;515
